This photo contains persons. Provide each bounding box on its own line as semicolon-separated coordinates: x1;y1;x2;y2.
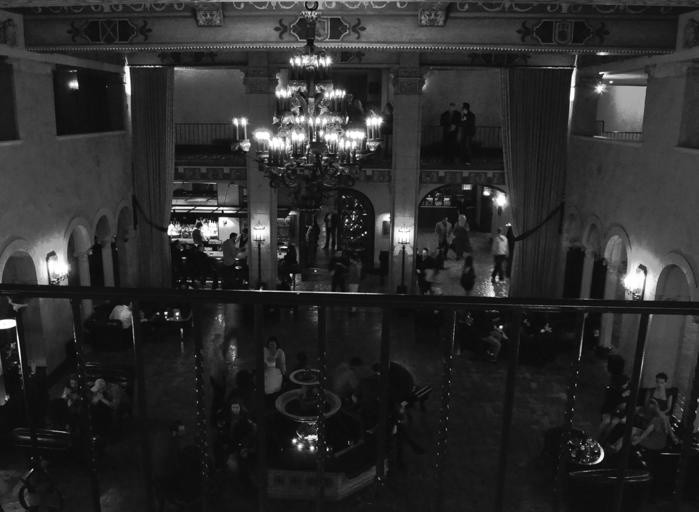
276;209;351;292
331;356;363;396
461;103;475;165
59;296;142;425
380;104;393;162
413;210;515;297
192;220;249;271
598;370;676;446
261;338;289;378
441;102;460;163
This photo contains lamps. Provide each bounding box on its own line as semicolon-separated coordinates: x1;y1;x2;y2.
0;306;16;330
45;250;67;282
620;265;647;297
230;7;385;213
